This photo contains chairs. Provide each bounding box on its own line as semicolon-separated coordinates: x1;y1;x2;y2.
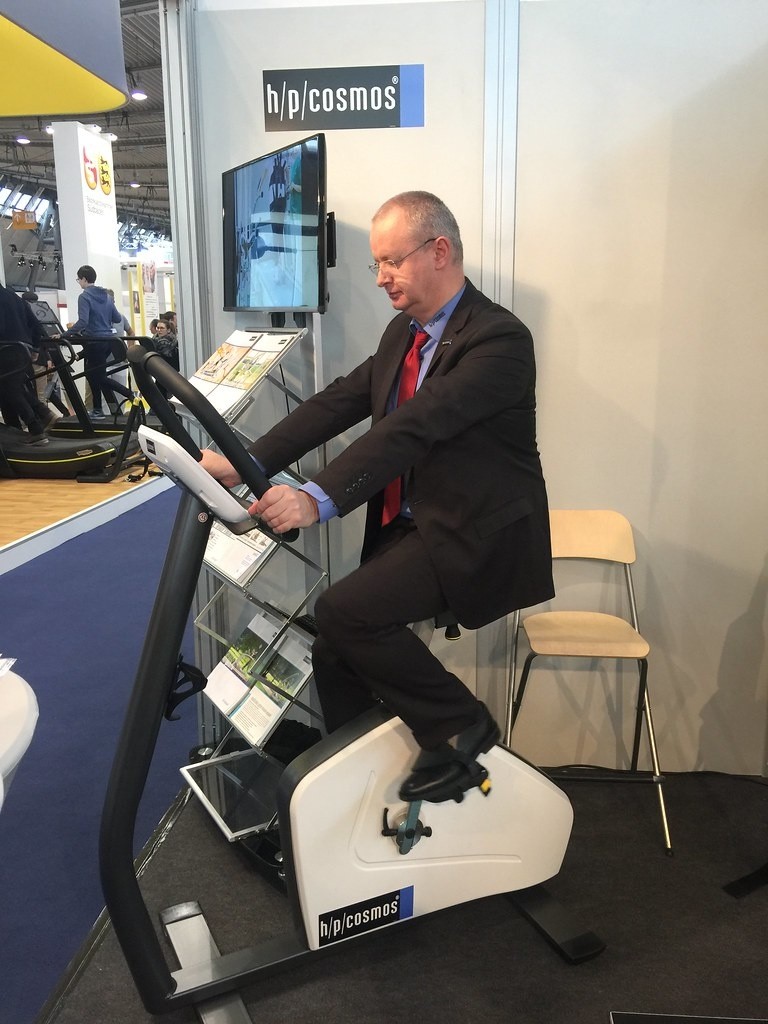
502;509;674;856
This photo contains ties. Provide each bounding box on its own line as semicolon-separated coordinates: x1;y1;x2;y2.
382;329;429;527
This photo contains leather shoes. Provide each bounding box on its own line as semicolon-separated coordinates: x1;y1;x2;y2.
400;702;501;803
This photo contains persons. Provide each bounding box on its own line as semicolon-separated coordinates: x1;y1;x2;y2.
202;192;554;803
50;265;140;420
102;288;135;415
149;311;179;400
0;284;60;445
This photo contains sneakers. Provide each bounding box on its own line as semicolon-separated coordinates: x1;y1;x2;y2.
17;412;59;446
88;410;107;420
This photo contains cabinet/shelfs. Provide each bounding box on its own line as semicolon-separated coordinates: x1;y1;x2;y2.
164;326;328;845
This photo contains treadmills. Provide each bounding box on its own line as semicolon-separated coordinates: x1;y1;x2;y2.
0;290;166;483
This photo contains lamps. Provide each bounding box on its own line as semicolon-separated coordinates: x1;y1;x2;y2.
131;150;140;188
131;38;148;100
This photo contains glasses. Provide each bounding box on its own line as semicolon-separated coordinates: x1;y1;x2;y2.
368;239;436;277
76;279;80;283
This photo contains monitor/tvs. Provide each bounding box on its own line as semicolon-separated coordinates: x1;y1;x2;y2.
221;133;329;313
